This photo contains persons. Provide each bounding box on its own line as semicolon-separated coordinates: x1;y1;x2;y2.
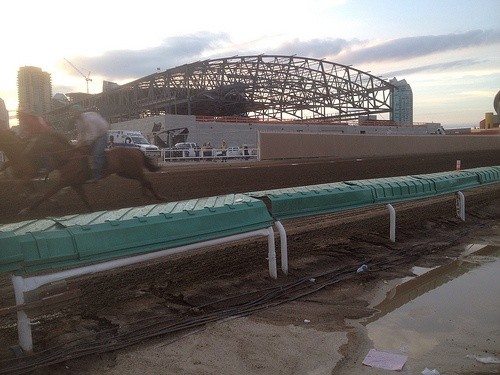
194;142;213;162
70;106;108;179
239;146;249;160
221;141;227;162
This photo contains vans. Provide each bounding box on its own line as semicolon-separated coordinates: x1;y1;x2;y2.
106;129;200;157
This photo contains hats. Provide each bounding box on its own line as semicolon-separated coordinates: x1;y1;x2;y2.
69;105;84;112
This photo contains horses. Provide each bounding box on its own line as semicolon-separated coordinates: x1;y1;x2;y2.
0;128;167;216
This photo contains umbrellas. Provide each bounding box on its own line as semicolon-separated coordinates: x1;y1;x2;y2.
239;144;247;146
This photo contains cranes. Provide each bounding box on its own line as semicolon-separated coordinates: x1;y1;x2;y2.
64;57;93;94
